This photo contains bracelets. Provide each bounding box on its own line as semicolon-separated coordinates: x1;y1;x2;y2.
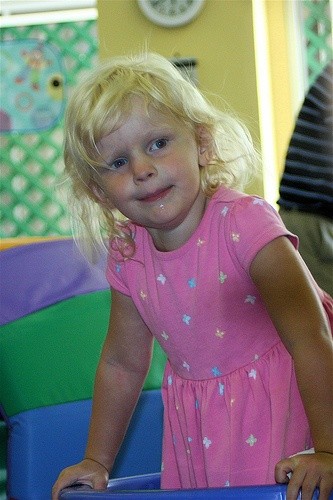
314;449;333;455
84;457;112;475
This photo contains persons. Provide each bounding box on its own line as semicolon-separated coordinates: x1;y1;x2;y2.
51;48;333;500
276;59;333;301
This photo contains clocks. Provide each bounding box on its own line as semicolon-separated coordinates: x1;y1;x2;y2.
137;0;203;28
